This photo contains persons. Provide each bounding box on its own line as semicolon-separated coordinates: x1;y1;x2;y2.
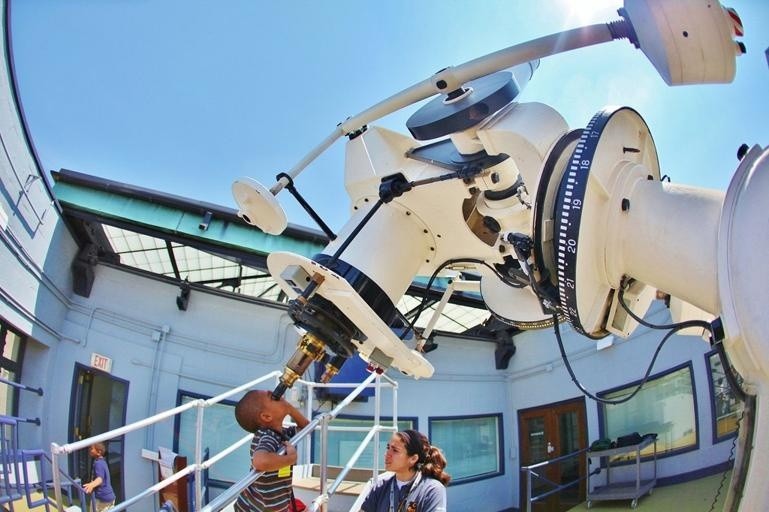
360;427;448;512
716;378;728;416
81;444;116;512
235;388;310;512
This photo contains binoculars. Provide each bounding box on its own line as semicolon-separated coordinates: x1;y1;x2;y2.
230;332;332;401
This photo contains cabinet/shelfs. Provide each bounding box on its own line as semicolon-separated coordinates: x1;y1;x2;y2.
585;434;660;510
